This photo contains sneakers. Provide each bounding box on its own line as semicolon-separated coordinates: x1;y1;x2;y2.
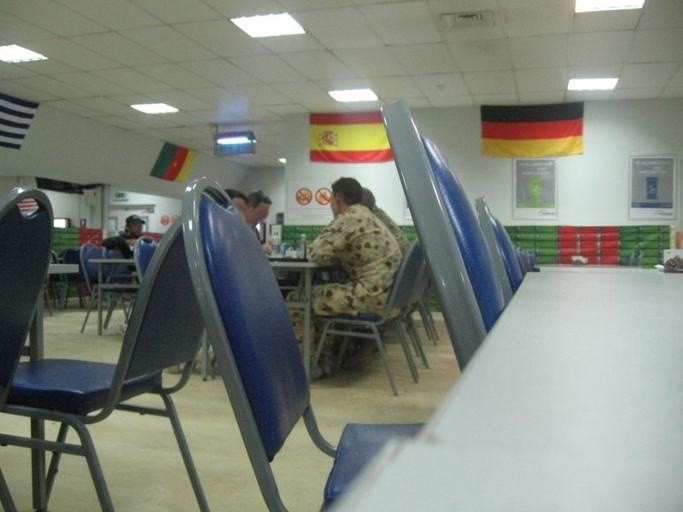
310;339;385;381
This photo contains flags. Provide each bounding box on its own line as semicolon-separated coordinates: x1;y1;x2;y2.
480;103;584;158
147;143;198;184
309;112;396;162
0;92;39;152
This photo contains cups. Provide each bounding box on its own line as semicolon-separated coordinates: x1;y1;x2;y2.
528;176;544;204
646;177;658;198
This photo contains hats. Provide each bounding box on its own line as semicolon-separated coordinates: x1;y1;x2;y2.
126;215;144;224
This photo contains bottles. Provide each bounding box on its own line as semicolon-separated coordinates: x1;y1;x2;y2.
270;231;308;258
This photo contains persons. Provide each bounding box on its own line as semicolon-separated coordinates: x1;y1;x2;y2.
247;188;273;255
282;176;414;381
225;189;247;217
101;214;152;286
342;186;411;370
